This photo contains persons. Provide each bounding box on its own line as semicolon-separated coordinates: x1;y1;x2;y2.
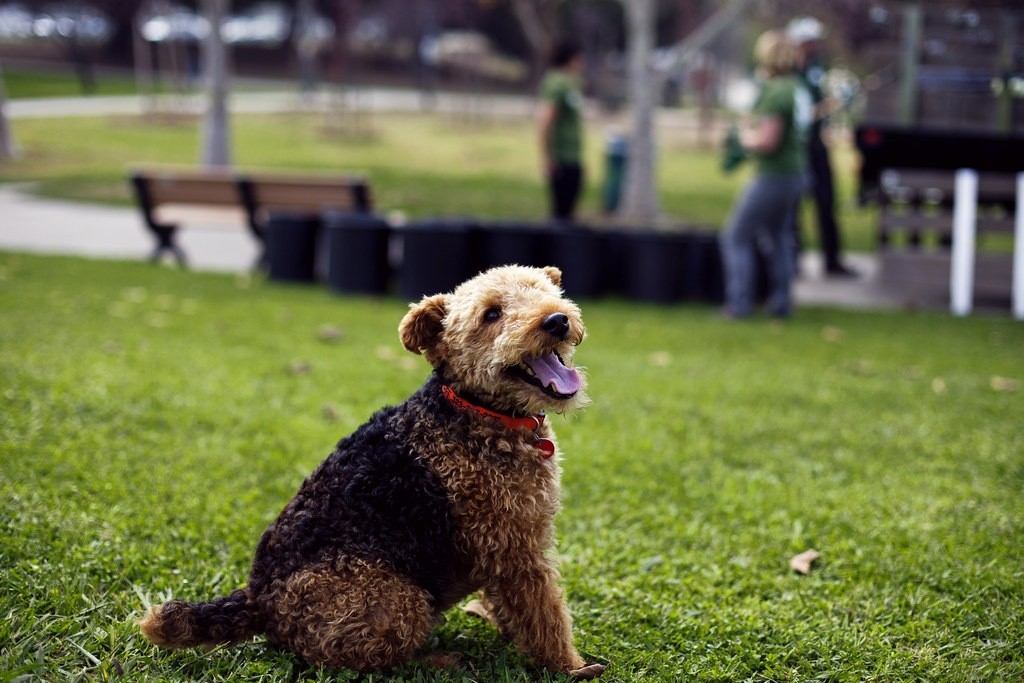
785;16;859;279
717;27;815;317
534;32;589;218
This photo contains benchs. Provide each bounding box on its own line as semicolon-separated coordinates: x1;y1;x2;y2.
884;175;1019;303
127;170;373;272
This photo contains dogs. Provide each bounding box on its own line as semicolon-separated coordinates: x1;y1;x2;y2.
140;263;608;676
